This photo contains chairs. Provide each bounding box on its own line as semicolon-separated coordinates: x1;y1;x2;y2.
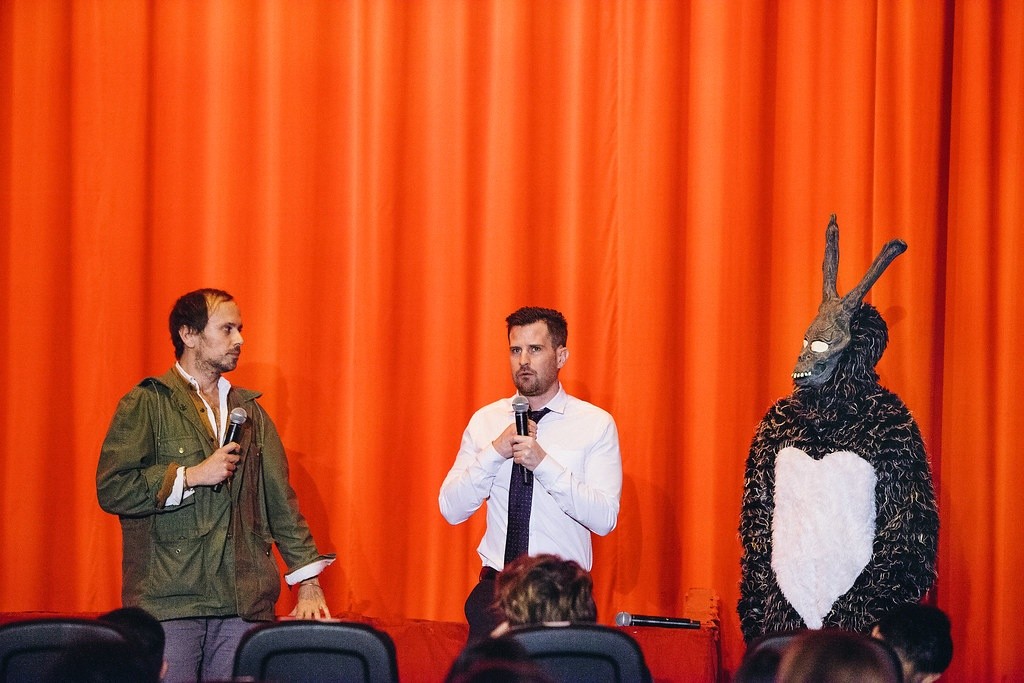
501;624;647;683
0;617;132;683
734;631;904;683
231;620;399;683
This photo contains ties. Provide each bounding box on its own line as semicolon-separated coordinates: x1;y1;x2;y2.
504;409;552;571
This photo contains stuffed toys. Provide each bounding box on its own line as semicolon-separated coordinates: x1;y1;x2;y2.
737;214;941;651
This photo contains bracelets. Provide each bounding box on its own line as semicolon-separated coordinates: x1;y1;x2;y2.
183;466;191;491
300;583;324;589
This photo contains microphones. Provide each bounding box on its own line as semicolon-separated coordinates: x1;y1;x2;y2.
209;407;248;494
513;396;531;483
614;611;700;630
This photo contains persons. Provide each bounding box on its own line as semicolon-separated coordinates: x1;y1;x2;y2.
441;554;598;683
771;602;955;683
49;605;169;683
94;288;331;682
437;307;623;643
